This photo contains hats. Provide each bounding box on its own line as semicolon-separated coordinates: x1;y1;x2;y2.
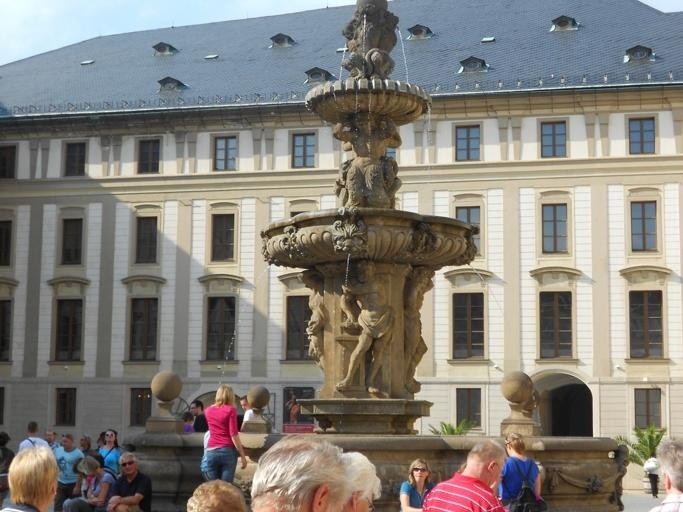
0;432;10;441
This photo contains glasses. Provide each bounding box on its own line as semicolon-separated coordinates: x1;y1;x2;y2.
121;461;133;466
412;467;425;472
366;497;376;512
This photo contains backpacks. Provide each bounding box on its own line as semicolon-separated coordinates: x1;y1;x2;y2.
503;458;547;512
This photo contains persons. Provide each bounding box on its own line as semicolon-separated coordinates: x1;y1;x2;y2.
644;453;660;498
403;267;434;391
336;260;394;394
648;436;683;512
297;269;326;372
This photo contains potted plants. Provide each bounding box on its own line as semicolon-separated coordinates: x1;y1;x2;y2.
614;421;668;495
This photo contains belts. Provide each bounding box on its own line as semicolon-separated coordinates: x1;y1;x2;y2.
0;473;9;477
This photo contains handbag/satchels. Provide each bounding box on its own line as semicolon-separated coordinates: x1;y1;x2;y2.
643;458;660;472
